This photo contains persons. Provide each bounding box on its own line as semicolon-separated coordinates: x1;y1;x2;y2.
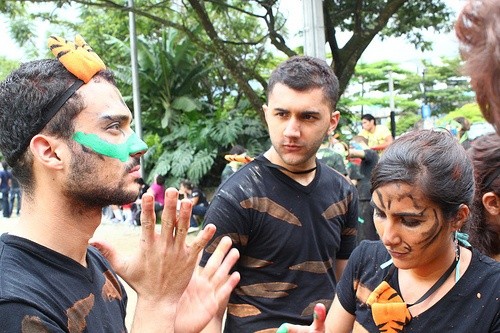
319;114;392;243
455;0;500;135
0;161;12;218
222;146;247;183
274;130;500;333
104;175;166;226
0;59;241;333
178;179;209;233
9;173;21;214
195;57;359;333
451;118;500;263
